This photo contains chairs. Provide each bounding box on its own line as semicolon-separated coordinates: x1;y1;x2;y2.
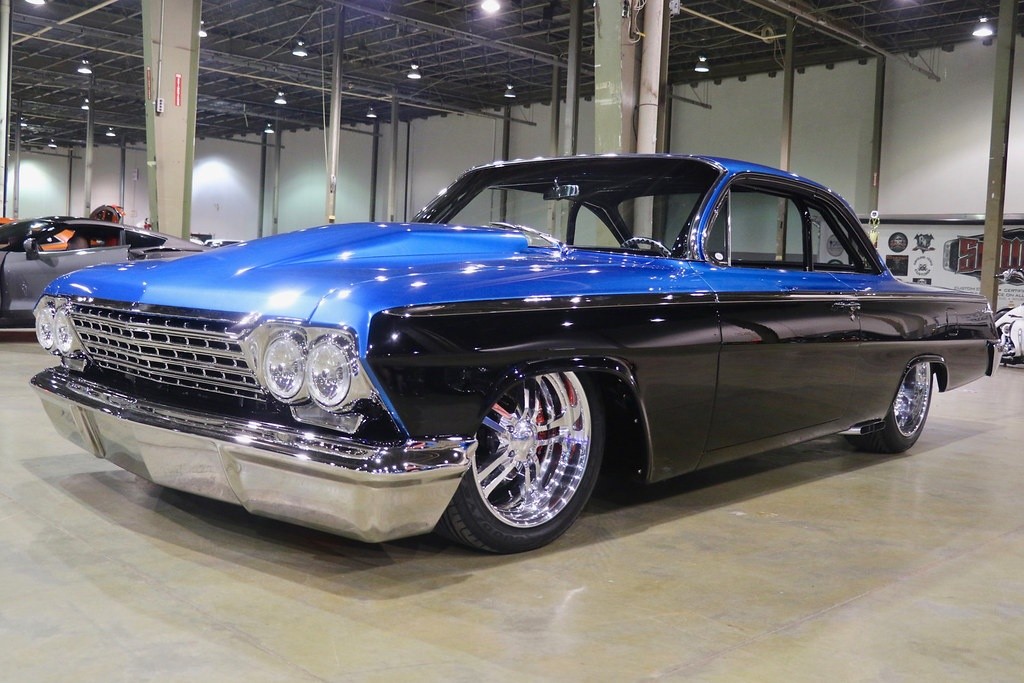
66;235;90;251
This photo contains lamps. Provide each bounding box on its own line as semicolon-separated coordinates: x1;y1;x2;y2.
77;58;93;74
694;54;711;73
407;62;422;79
366;106;378;118
503;83;516;98
274;88;287;105
105;127;117;137
263;121;275;134
292;37;309;57
47;139;58;149
199;20;208;38
20;117;28;127
973;14;994;37
81;99;90;111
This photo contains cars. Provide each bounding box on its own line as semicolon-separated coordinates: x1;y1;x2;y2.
0;213;248;328
991;303;1024;372
27;150;990;553
